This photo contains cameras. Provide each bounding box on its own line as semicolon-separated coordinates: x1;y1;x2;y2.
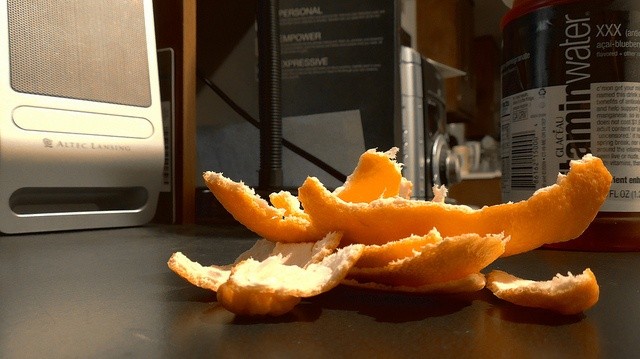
400;46;463;199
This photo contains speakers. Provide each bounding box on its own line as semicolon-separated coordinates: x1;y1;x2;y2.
0;0;166;234
